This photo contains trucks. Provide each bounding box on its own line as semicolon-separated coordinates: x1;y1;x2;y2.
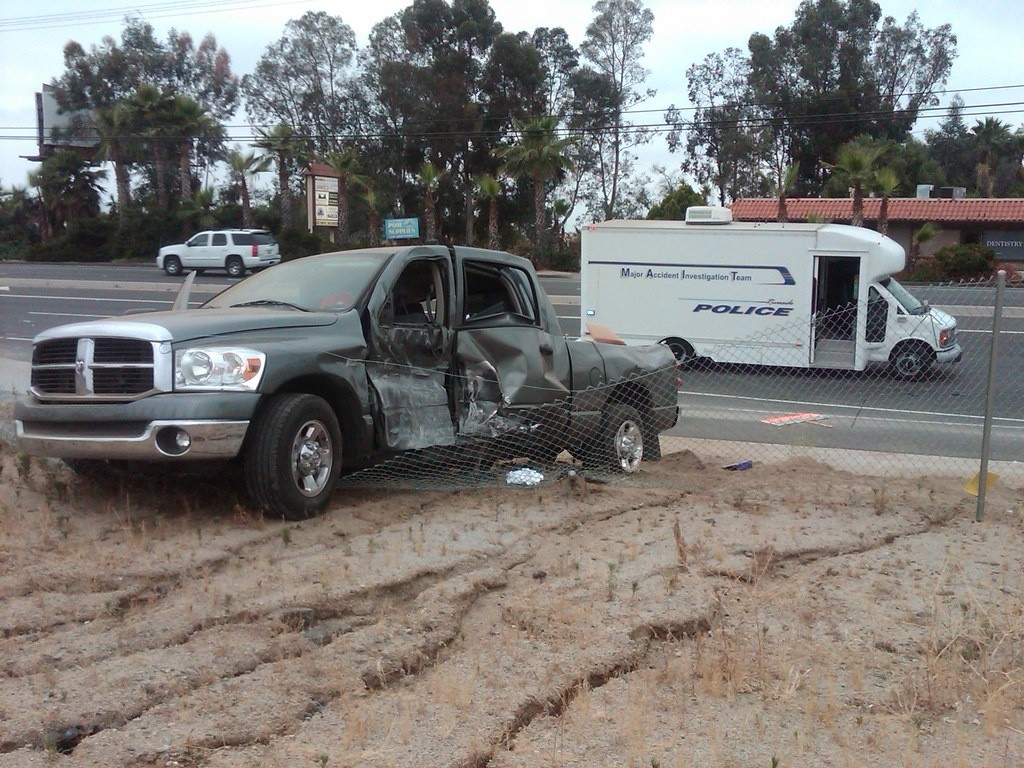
575;205;963;381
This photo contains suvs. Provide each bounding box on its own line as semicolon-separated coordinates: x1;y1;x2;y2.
157;227;282;278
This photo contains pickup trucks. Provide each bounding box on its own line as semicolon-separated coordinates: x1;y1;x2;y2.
12;244;683;522
